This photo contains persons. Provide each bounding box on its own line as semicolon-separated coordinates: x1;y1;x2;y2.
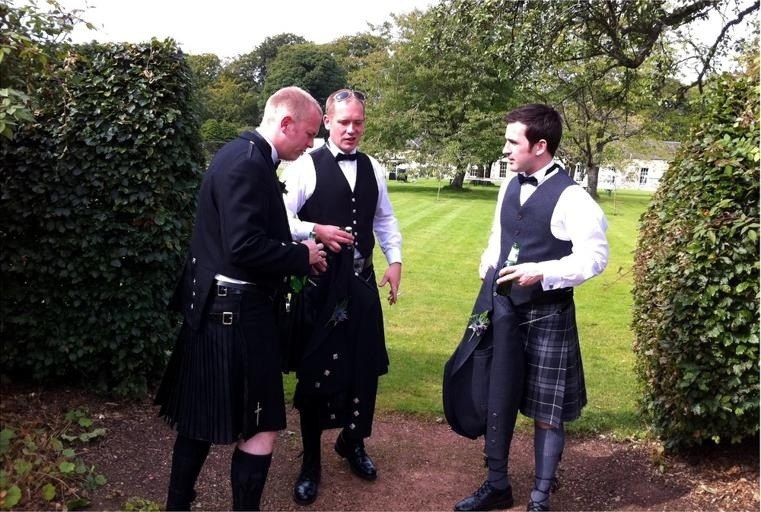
277;88;407;505
149;86;329;511
442;102;612;511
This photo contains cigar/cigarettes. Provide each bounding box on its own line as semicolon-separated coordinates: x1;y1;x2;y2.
387;290;404;300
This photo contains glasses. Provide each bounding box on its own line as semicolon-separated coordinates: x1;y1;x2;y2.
333;91;364;102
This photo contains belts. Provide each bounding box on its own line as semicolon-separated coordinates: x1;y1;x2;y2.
198;284;270;327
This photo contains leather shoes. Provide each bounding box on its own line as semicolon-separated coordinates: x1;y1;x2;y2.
294;470;318;505
334;432;377;481
455;480;513;511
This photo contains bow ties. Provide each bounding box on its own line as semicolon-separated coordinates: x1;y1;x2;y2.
518;173;538;186
335;153;356;161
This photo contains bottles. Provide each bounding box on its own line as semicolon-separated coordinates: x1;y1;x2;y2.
495;241;520;295
287;230;318;292
346;226;355;251
